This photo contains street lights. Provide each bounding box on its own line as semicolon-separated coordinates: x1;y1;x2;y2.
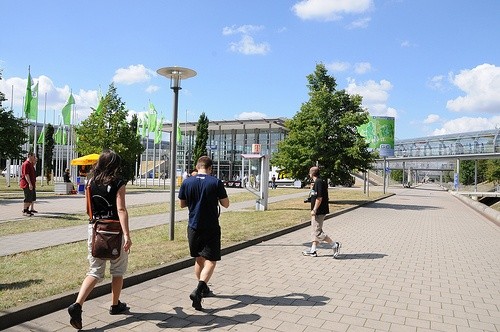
161;155;168;188
155;66;198;241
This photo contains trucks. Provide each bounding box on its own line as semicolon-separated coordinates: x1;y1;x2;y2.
1;164;22;178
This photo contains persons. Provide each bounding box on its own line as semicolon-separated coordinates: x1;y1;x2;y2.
67;149;132;329
302;167;339;258
45;165;51;185
149;171;167;178
78;165;86;176
178;155;229;310
191;169;197;175
181;168;187;181
64;167;75;190
233;172;260;188
271;175;277;190
20;153;38;216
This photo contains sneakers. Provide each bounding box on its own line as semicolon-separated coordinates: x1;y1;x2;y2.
331;241;342;258
190;289;202;311
68;303;83;329
302;250;317;257
109;300;127;315
197;283;210;297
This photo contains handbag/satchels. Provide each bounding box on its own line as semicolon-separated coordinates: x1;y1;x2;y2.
92;218;123;260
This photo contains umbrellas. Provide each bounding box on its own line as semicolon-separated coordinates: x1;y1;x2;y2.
70;153;102;166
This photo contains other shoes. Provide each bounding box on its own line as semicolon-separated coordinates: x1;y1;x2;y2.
31;213;34;215
22;211;31;217
30;210;37;213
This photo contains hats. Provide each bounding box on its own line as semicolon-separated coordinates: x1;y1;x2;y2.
65;168;70;172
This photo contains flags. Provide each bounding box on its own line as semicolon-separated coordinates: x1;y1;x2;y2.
136;112;165;144
38;125;70;145
62;94;75;126
177;119;182;144
24;75;37;120
148;103;157;133
